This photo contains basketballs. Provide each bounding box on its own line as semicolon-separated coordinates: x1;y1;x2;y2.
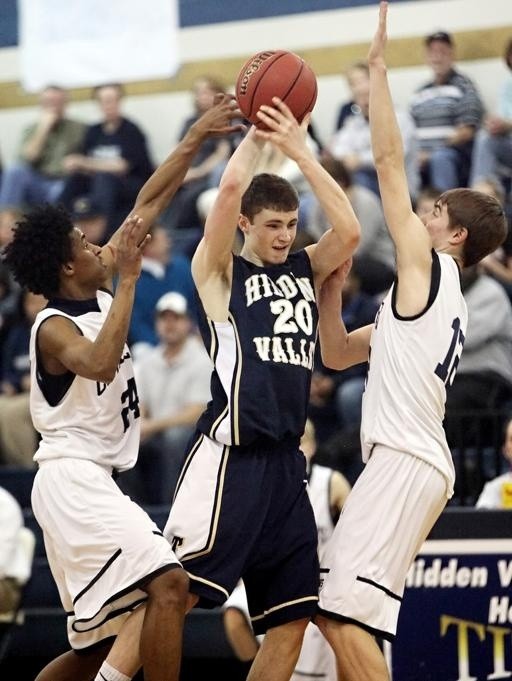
236;49;317;130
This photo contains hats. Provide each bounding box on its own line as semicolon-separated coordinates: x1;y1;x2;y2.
425;31;451;47
155;291;190;314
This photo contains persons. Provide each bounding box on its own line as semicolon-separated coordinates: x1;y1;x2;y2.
1;0;512;681
